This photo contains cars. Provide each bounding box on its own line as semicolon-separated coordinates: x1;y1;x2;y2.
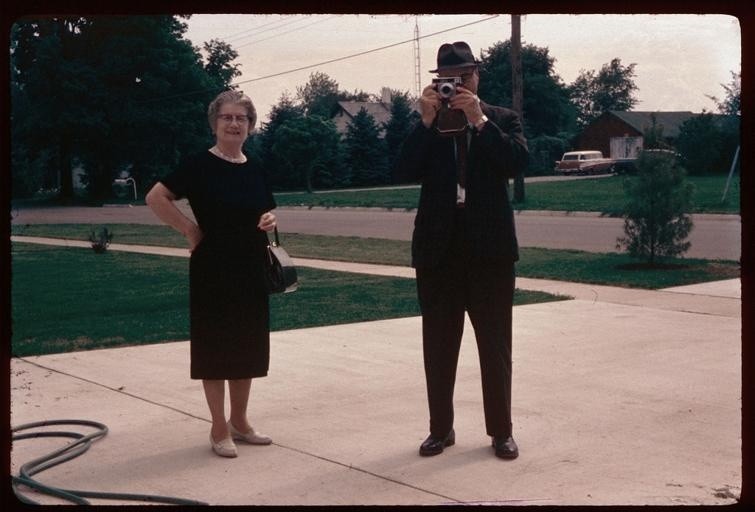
554;150;604;175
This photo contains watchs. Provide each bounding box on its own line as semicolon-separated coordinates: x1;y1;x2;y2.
473;116;488;128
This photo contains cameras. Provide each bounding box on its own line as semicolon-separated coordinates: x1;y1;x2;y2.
432;77;464;98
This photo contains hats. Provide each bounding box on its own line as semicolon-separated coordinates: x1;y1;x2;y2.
429;41;481;73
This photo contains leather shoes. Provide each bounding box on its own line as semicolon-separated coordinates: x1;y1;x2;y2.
229;423;272;442
210;428;237;457
488;434;518;458
418;430;456;454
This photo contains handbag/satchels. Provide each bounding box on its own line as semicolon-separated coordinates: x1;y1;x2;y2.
267;246;298;293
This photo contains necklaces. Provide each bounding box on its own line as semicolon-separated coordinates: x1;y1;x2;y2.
213;148;246;163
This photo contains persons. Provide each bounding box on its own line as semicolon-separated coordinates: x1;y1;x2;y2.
145;92;277;457
396;41;530;460
